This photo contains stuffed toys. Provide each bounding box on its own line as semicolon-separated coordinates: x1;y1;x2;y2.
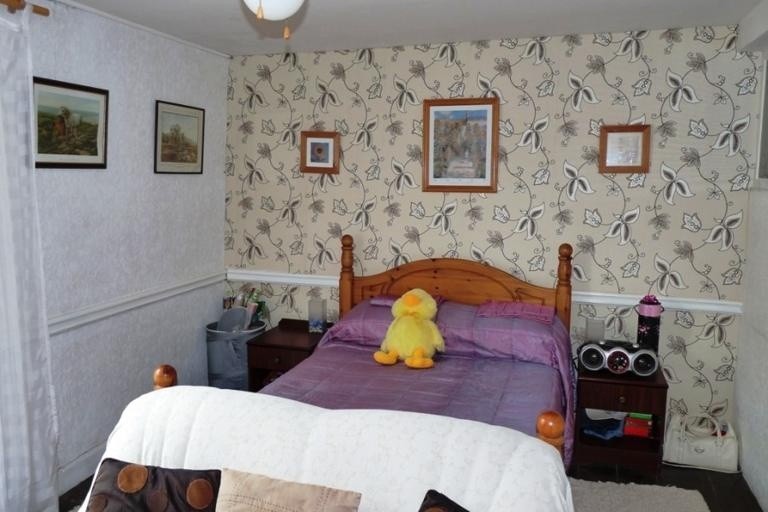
373;288;445;369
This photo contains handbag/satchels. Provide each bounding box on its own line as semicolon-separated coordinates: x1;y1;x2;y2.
662;409;742;476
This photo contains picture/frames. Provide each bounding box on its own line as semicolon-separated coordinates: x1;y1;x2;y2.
22;76;109;170
299;131;340;174
598;124;651;174
422;96;500;194
153;100;205;175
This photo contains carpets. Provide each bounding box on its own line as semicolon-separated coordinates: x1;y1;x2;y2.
566;476;711;512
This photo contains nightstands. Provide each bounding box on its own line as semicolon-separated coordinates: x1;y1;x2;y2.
246;318;335;392
572;339;670;483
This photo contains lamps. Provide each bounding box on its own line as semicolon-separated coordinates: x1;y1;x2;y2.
242;0;305;22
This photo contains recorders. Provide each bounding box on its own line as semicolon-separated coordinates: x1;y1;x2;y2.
576;338;659;378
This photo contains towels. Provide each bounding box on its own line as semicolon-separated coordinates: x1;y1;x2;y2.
477;300;556;325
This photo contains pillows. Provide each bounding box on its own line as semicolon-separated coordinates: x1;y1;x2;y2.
435;300;569;369
316;291;437;349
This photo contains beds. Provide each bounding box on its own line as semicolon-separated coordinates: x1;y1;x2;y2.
76;234;575;512
